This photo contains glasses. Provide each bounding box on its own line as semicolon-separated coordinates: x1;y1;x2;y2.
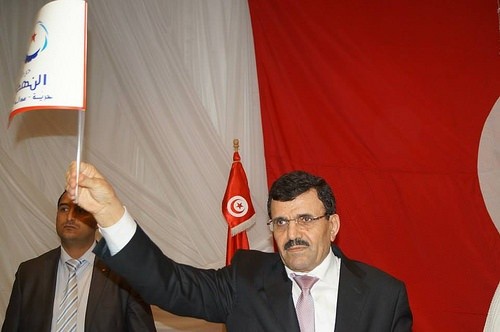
266;210;329;233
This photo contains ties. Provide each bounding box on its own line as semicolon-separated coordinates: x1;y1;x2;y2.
56;258;89;332
292;272;320;332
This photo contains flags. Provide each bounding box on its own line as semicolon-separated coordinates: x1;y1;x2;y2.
7;2;87;125
221;152;259;264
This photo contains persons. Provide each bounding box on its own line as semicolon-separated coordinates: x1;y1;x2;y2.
0;178;160;332
68;158;414;332
